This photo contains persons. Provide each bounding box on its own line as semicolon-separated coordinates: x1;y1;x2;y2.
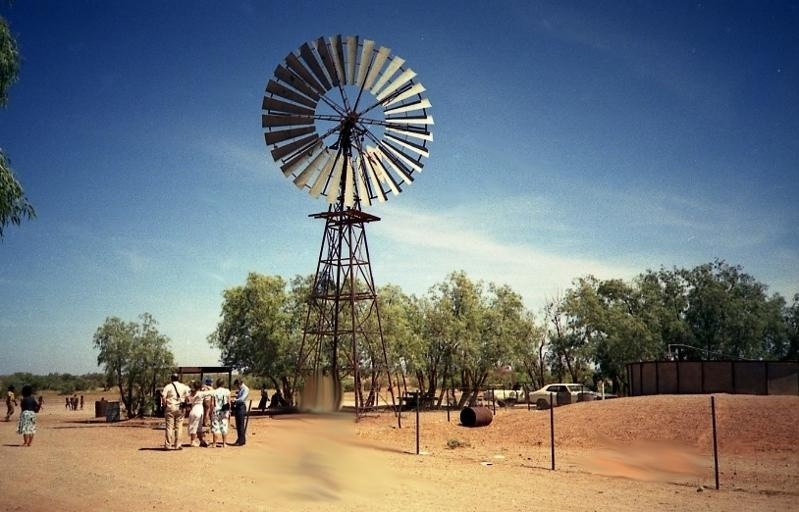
162;372;250;449
154;390;163;415
258;383;282;409
15;386;41;447
65;395;83;410
5;386;18;421
512;382;536;400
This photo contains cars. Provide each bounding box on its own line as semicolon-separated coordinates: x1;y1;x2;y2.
529;383;597;410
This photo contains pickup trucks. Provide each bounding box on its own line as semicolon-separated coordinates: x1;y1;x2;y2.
484;384;534;407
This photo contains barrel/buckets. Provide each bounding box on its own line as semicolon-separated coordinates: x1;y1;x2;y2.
105;401;120;423
459;407;493;426
95;400;108;417
